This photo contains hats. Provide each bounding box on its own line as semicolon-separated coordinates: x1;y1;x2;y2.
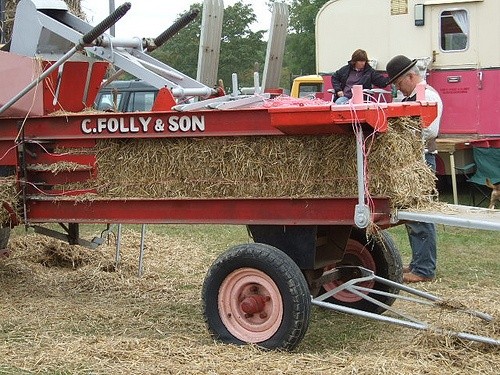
385;54;418;86
352;48;369;61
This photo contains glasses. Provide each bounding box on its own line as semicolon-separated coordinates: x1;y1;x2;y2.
391;72;409;87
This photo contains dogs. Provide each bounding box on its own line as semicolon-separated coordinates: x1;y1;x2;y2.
486;176;500;209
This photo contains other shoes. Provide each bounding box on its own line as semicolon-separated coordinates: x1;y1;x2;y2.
402;266;414;273
403;272;434;282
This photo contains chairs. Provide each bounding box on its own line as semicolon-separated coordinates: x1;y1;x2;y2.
454;147;500;208
327;57;433;104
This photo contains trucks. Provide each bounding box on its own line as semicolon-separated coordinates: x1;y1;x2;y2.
314;0;500;193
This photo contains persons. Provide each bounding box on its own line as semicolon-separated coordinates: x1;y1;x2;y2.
384;55;443;283
331;50;391;105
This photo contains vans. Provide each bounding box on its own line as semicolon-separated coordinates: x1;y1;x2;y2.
93;80;198;112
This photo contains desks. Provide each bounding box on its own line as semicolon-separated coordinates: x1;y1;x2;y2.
435;137;476;205
